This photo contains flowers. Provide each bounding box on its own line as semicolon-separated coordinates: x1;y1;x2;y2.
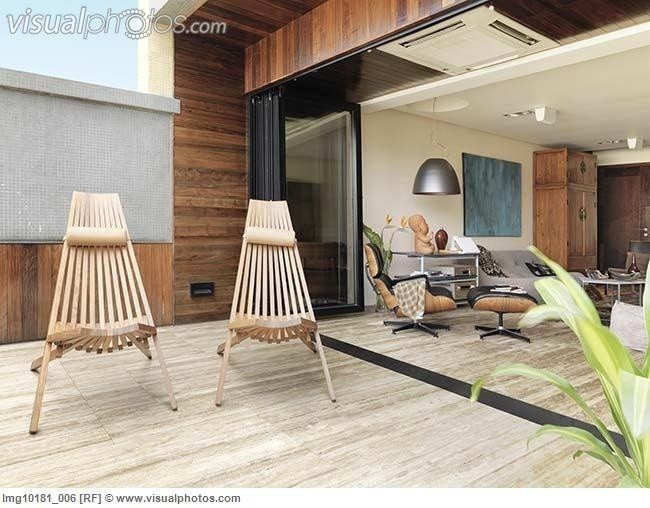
363;212;408;284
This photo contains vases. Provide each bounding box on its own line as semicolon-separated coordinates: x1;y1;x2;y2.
372;282;389;317
434;226;448;250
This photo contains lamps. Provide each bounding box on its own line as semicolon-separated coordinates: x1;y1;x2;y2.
410;96;467;196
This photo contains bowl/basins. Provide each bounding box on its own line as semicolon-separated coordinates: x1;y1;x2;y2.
610;267;639;280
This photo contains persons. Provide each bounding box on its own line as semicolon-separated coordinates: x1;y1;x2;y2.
408;215;435;254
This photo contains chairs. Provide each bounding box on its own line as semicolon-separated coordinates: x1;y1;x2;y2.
608;240;650;296
364;237;459;337
214;190;337;410
29;191;179;438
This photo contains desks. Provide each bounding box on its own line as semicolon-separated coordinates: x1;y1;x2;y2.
577;271;647;322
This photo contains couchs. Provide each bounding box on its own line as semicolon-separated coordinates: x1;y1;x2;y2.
477;238;586;310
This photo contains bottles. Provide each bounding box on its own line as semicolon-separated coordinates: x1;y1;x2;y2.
435;225;448;250
627;253;640;274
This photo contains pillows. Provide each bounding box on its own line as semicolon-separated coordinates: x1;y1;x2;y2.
625;251;649;275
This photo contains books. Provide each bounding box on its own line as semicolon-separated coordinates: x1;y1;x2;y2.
490;286;528;294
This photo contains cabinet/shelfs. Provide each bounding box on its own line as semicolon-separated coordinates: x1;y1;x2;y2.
531;146;598;271
387;242;482;312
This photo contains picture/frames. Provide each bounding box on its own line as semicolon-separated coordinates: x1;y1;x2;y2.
462;152;522;238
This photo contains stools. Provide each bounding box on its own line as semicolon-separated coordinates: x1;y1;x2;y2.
466;285;539;344
